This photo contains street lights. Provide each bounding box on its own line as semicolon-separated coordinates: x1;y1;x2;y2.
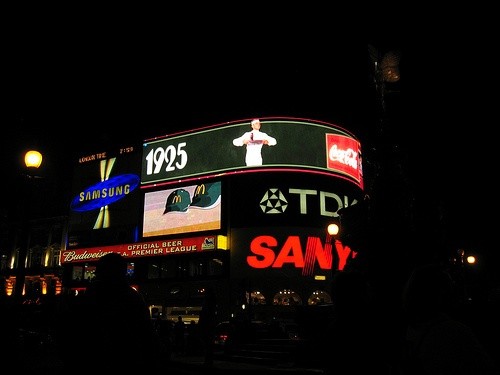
17;149;46;268
327;224;341;278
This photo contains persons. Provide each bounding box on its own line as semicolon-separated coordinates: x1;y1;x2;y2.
65;254;149;375
292;272;404;375
233;118;277;167
